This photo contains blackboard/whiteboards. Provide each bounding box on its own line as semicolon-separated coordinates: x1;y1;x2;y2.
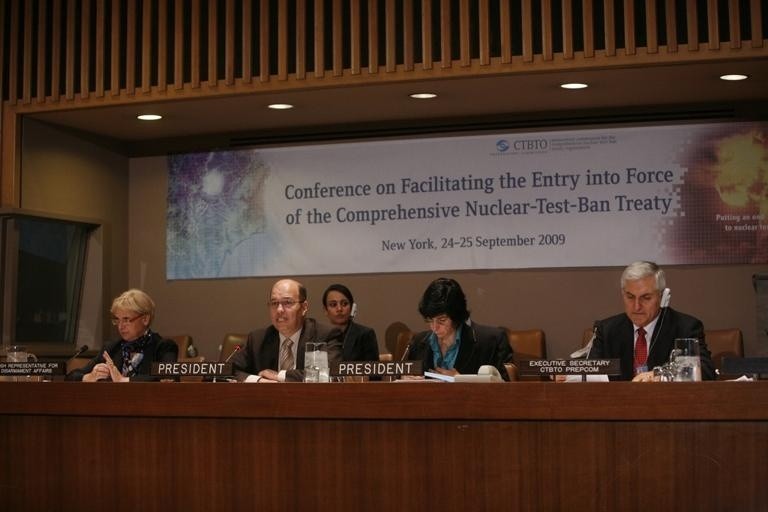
455;365;507;383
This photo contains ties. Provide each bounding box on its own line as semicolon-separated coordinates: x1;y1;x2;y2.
110;314;144;325
633;326;648;376
280;337;295;371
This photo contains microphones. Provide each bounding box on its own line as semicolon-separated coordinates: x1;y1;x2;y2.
582;320;601;382
212;343;245;383
389;339;415;379
50;344;89;382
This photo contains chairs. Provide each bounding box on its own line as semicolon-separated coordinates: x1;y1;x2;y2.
700;326;744;381
582;329;594;349
219;330;250;371
752;270;767;334
164;336;192;382
500;329;547;383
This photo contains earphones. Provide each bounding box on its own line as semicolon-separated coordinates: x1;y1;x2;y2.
660;288;671;308
350;303;357;317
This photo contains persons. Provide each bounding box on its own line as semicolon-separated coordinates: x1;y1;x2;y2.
231;278;345;383
392;277;514;382
64;289;179;383
585;261;716;382
324;284;382;381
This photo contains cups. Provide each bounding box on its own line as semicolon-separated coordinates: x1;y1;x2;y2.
654;338;703;382
5;344;28;361
305;339;331;382
